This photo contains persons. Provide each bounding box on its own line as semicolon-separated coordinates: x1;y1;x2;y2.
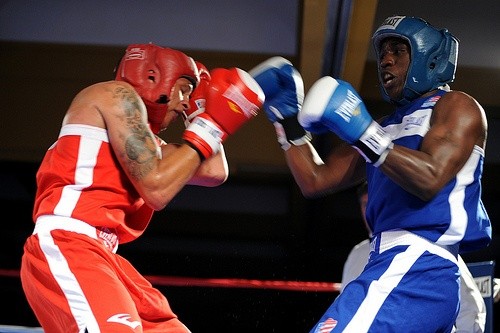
247;15;492;333
19;43;265;333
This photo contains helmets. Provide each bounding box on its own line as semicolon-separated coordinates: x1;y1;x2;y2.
371;16;459;100
115;44;199;135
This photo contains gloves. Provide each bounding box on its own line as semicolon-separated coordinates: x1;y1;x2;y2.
249;56;311;150
182;67;265;160
180;62;214;128
298;76;392;166
